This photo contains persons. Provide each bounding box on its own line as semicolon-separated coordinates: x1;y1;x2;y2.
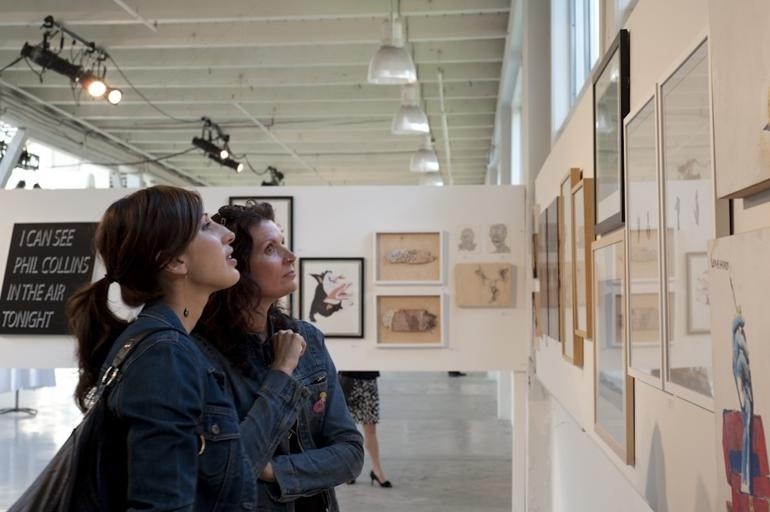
338;372;392;488
190;202;365;512
64;186;258;512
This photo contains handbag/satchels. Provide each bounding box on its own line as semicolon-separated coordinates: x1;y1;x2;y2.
6;326;206;512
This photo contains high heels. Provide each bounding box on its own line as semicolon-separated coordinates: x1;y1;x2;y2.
370;471;392;487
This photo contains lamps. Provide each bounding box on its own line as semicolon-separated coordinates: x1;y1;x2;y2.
21;15;123;106
367;0;444;186
192;116;244;173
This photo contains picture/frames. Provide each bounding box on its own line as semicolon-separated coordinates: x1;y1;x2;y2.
229;195;294;319
373;231;445;286
373;289;446;348
298;257;365;339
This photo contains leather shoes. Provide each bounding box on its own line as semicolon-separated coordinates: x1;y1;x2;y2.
450;372;465;376
